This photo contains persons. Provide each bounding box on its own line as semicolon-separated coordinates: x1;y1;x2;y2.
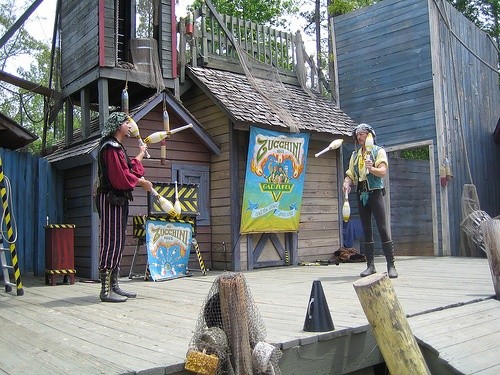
342;123;398;278
95;112;153;303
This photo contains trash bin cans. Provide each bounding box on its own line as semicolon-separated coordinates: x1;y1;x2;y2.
44;222;76;286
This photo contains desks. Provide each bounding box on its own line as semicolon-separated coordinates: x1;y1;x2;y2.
128;211;207;281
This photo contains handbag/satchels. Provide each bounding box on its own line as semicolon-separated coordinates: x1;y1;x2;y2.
105;191;125;207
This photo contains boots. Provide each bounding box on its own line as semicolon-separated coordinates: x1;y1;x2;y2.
360;242;376;277
381;240;398;278
100;268;128;302
110;269;136;298
